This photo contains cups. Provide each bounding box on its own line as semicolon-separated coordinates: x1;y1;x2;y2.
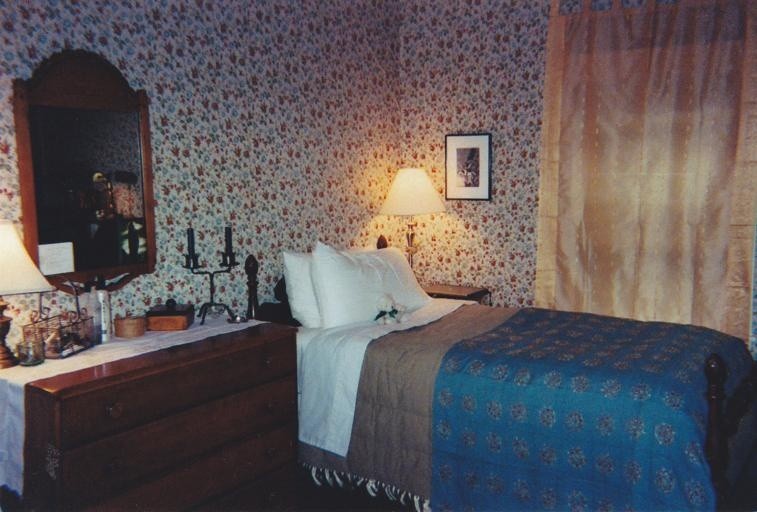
16;339;44;366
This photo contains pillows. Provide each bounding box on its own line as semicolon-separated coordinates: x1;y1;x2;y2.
309;238;432;332
281;250;317;332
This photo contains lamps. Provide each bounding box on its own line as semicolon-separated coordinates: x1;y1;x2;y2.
1;225;51;367
378;167;445;269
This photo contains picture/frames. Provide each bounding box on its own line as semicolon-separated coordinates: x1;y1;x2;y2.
445;133;491;201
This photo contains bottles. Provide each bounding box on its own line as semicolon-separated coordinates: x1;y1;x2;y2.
85;286;103;345
97;290;110;342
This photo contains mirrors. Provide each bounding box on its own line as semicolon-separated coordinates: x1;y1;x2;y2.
9;46;156;292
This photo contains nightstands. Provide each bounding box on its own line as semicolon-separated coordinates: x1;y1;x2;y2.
424;285;489;306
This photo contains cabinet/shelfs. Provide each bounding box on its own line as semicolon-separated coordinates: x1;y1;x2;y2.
0;314;299;511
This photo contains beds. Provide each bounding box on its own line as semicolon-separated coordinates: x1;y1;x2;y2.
243;235;755;510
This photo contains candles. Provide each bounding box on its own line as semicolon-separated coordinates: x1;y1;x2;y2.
222;225;231;248
184;226;195;251
